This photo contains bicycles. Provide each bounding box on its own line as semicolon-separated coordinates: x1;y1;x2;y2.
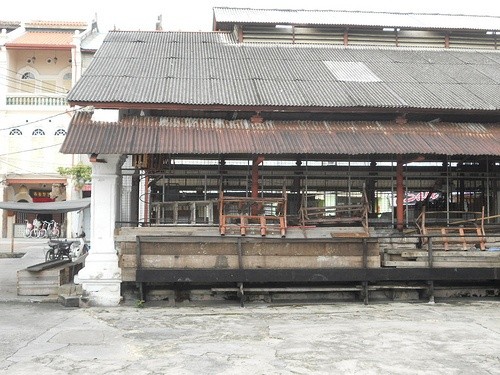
45;243;73;263
23;219;59;238
